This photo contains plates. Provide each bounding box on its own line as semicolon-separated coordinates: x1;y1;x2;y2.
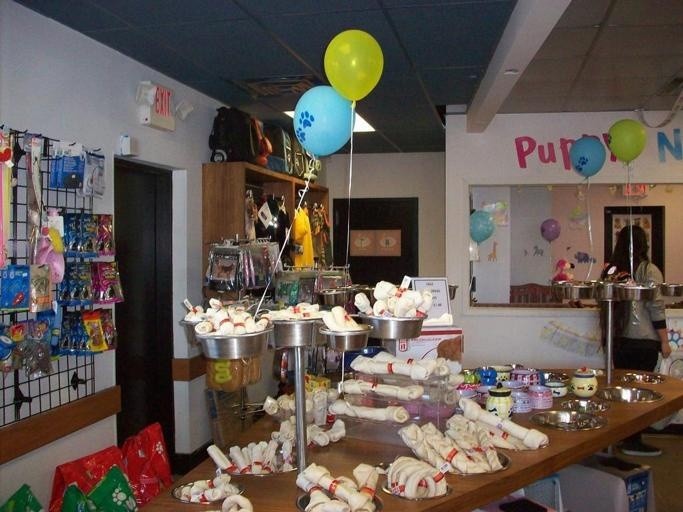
560;398;609;412
443;448;510;474
596;387;662;403
618;373;667;385
215;452;300;476
383;478;452;499
528;408;606;431
171;480;245;503
296;491;383;512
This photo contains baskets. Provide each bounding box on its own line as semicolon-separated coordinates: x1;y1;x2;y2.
510;283;565;303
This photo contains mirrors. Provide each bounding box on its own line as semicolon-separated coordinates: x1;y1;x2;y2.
469;184;683;307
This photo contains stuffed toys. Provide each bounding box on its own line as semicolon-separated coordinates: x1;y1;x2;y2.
550;259;575;282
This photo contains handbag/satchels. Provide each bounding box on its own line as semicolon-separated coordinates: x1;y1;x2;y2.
213;107;318;183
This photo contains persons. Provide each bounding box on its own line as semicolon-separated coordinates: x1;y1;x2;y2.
592;226;671;459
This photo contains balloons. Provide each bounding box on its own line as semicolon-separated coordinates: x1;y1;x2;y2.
605;118;648;165
468;209;494;243
322;30;384;102
292;85;356;159
540;218;561;241
567;132;606;179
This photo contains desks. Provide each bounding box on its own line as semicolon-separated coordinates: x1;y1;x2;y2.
134;367;683;512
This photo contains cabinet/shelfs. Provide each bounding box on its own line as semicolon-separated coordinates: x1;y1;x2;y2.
202;161;330;300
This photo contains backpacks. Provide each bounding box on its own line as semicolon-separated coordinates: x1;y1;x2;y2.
600;256;647;332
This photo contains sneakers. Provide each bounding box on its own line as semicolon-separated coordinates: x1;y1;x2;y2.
621;439;662;456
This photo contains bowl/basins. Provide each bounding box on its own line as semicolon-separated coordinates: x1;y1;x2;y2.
565;285;591;299
355;313;422;339
195;322;273;360
319;325;368;350
660;284;683;296
554;285;565;300
340;346;387;370
272;320;314;350
319;287;345;309
458;363;567;413
595;283;616;299
616;285;657;301
180;319;202;346
